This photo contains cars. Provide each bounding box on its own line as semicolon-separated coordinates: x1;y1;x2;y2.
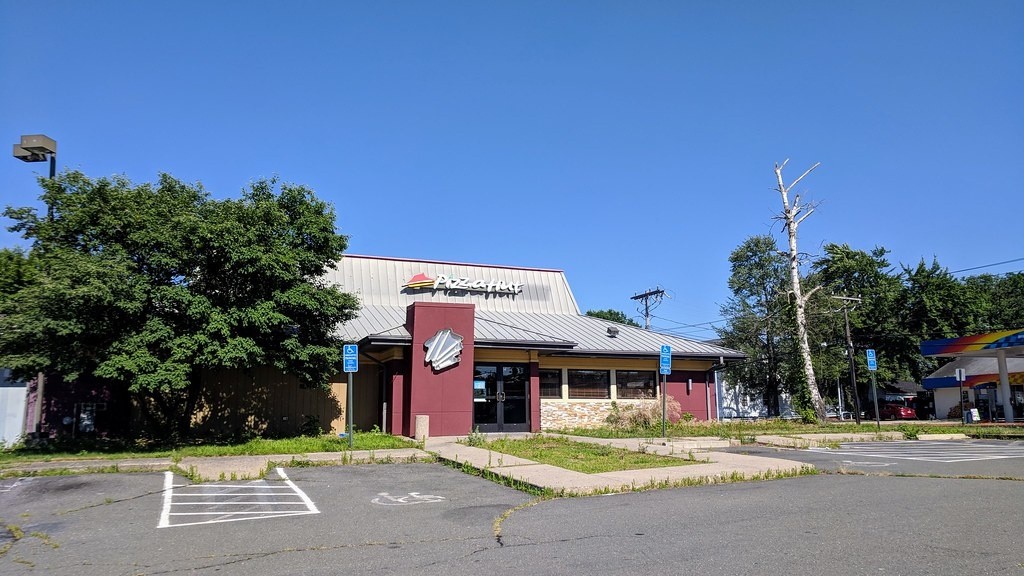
880;403;917;420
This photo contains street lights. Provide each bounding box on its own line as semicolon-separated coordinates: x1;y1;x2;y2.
14;134;57;224
